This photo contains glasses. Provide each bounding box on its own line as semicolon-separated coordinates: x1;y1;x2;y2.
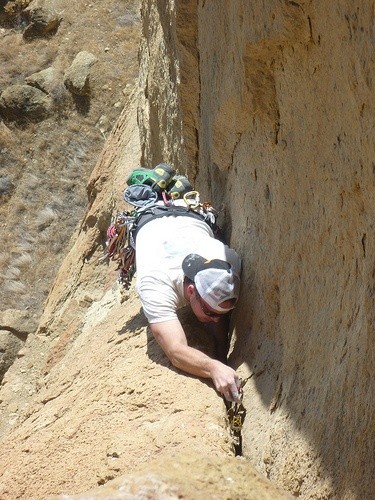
194;287;230;317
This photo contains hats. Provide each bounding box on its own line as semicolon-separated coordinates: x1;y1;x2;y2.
182;253;240;312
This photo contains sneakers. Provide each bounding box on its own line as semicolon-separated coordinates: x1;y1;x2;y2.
159;175;192;199
123;164;176;194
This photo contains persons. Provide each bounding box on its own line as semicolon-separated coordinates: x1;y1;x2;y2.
128;199;243;404
